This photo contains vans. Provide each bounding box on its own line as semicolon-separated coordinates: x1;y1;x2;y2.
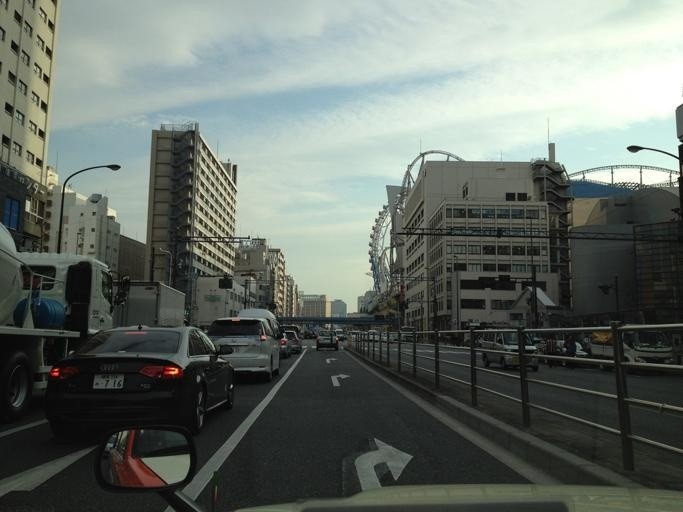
480;328;538;371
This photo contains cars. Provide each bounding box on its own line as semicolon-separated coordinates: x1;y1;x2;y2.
532;337;590;369
192;307;416;381
108;430;190;488
41;323;235;439
104;436;116;454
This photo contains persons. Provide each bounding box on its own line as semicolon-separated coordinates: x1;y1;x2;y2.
545;334;575;368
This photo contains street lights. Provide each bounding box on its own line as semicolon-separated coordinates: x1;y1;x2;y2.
625;142;681;222
55;164;122;253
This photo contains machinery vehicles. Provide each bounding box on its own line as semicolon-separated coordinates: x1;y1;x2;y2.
0;223;116;419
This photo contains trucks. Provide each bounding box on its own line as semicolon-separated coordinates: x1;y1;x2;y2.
111;278;186;329
589;328;675;376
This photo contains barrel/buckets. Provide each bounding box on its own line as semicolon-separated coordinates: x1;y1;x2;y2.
14;299;69;327
